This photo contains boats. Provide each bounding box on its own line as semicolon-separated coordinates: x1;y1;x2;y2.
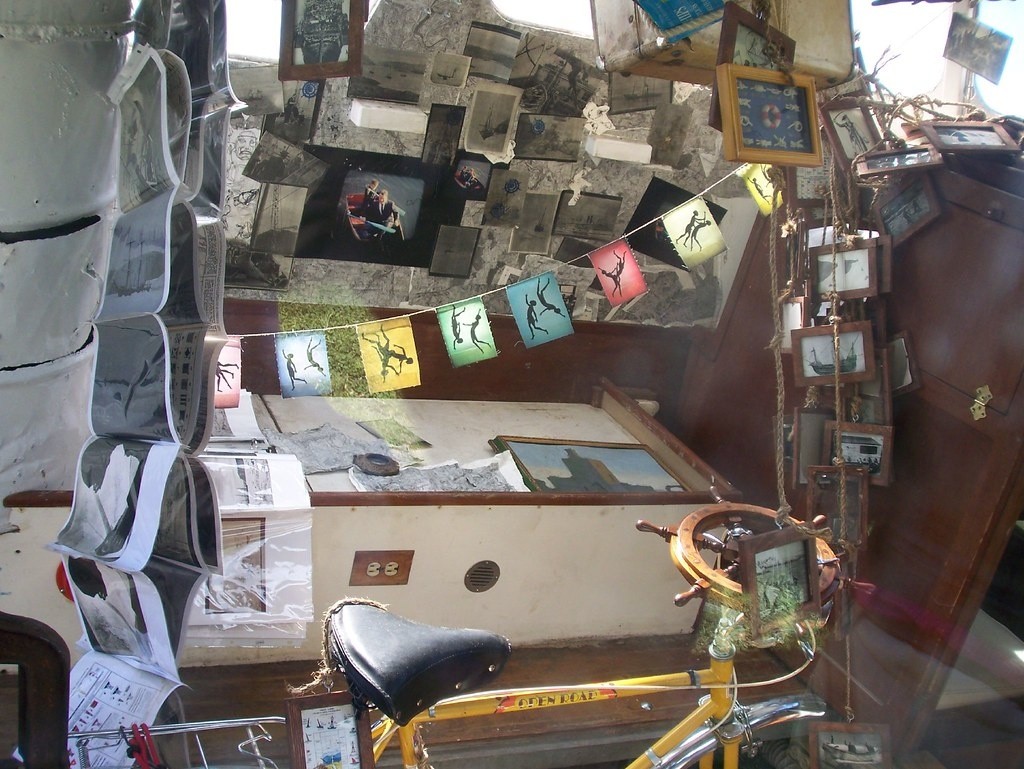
346;193;404;258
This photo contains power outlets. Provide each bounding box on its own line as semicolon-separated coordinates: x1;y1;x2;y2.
349;550;416;586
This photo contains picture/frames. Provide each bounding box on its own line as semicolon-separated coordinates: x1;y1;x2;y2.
816;101;886;180
779;295;807;353
809;721;892;769
494;434;694;492
856;143;945;179
277;1;370;82
446;149;496;202
790;320;876;387
804;296;894;426
716;63;823;168
803;207;833;230
739;519;822;639
791;407;837;491
809;238;879;304
786;139;847;209
292;143;467;269
286;690;376;769
708;1;797;131
806;465;870;555
820;420;895;487
878;234;894;296
871;171;942;250
887;328;921;398
918;121;1023;153
943;12;1014;85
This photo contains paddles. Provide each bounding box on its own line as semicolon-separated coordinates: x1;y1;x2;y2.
368;186;405;216
347;212;396;234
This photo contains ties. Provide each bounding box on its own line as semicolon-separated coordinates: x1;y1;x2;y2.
381;204;383;213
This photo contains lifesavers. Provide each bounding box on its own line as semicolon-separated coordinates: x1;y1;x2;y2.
761;103;781;129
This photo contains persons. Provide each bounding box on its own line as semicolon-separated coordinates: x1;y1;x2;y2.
258;150;289;181
567;68;589;94
833;113;869;153
757;578;770;611
284;93;304;126
460;166;473;186
357;179;400;240
903;200;920;223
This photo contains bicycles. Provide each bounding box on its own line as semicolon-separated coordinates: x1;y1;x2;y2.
69;486;876;768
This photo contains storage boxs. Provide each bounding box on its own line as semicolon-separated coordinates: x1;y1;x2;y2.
590;0;859;93
